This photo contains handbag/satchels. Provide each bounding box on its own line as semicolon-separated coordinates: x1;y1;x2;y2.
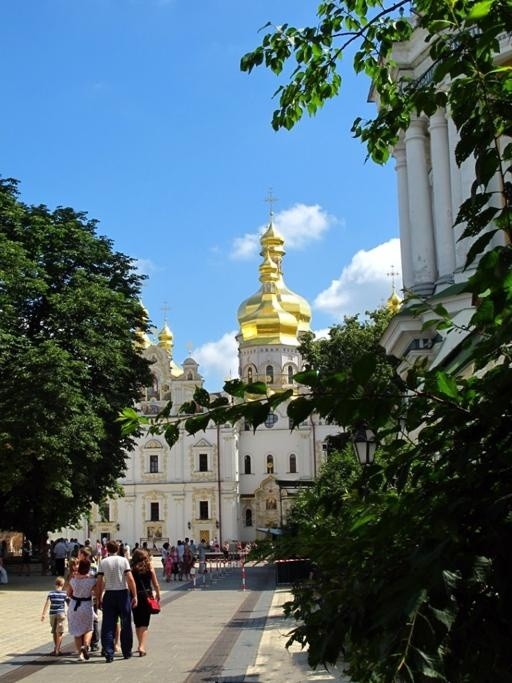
146;596;162;615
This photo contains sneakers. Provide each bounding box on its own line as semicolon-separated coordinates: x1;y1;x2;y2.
79;654;87;661
80;644;91;660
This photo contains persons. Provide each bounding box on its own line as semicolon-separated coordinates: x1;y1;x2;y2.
39;576;70;655
245;540;251;548
17;538;33;577
235;541;242;558
251;541;258;549
223;540;231;561
62;540;160;664
43;535;219;584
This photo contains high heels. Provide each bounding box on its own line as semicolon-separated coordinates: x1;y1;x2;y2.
137;650;146;657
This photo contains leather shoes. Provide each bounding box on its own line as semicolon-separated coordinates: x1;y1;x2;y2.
104;657;114;663
91;643;99;652
